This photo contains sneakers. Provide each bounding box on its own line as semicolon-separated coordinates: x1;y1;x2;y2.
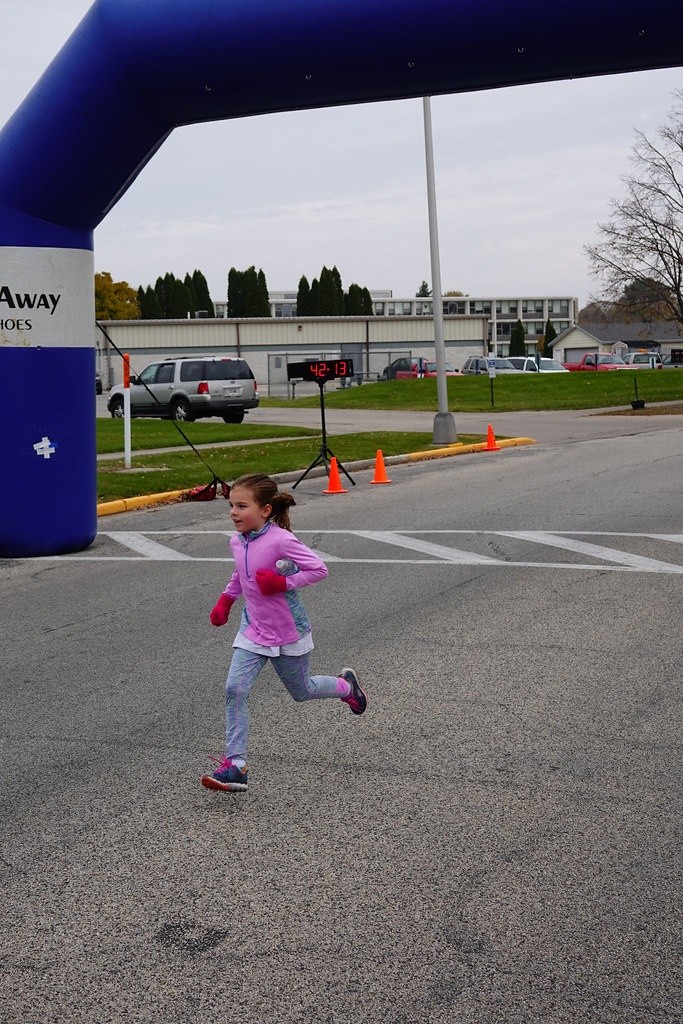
339;668;367;715
201;754;248;792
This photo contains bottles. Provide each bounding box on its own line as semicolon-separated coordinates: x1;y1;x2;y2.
276;557;300;576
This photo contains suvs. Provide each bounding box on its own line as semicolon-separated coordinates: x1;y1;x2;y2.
106;355;259;423
461;355;528;375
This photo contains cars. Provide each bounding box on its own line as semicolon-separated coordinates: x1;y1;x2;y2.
96;368;103;395
502;356;571;373
383;356;428;381
395;360;464;380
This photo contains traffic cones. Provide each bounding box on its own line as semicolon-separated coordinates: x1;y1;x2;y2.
321;456;348;494
482;423;502;451
368;449;392;484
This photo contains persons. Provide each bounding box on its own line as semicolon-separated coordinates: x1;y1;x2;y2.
201;472;368;793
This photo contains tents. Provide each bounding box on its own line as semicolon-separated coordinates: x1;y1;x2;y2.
612;340;662;353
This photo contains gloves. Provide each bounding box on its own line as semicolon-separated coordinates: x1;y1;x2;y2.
254;568;287;597
210;593;233;626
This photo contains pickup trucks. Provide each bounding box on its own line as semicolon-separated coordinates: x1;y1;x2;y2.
559;352;640;371
621;351;683;370
